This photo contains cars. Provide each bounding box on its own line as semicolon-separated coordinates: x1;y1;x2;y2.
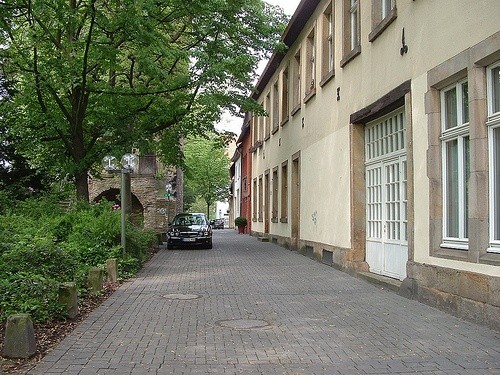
211;218;224;229
166;212;215;250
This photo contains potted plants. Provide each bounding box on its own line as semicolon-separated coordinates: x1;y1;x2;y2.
235;217;247;234
